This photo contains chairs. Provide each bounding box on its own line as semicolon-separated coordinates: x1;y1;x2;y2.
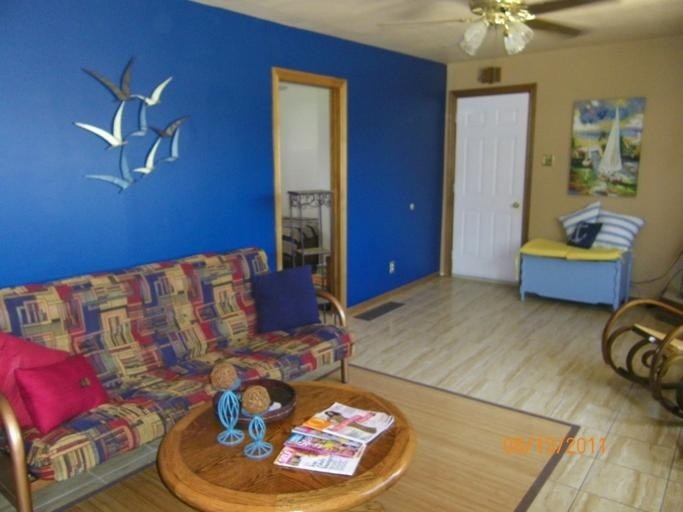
601;298;683;420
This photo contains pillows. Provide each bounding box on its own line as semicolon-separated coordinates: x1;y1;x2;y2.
0;331;71;429
555;201;601;242
250;265;321;336
590;208;647;251
567;221;604;249
13;353;111;434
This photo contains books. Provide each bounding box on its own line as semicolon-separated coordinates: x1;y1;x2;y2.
272;401;395;478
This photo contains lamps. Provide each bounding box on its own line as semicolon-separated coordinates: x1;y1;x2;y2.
458;0;535;59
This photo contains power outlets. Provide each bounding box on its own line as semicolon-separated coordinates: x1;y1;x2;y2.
390;262;395;273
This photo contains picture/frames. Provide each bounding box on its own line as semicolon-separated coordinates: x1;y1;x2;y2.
566;96;647;198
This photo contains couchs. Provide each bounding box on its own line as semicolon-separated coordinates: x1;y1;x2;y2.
0;249;353;512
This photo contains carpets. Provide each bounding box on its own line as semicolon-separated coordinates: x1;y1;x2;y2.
52;360;582;512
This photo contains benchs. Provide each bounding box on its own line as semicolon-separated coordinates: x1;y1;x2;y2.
519;238;632;312
